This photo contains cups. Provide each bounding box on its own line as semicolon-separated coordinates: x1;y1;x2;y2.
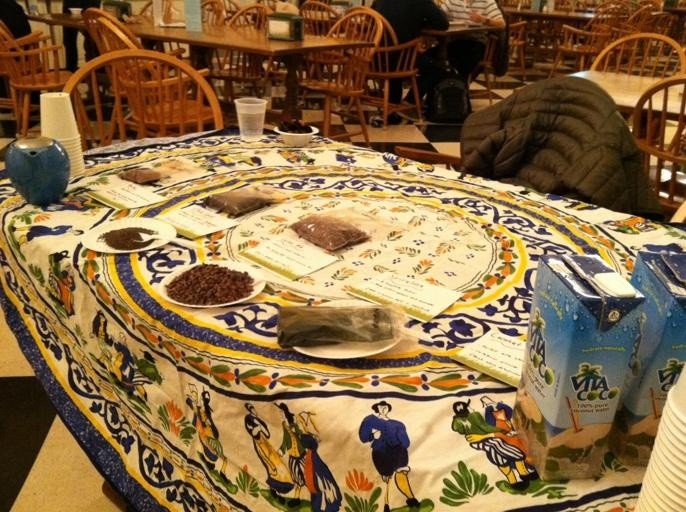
633;360;686;512
234;96;267;144
39;91;88;177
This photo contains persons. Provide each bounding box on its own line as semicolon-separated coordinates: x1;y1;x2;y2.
1;1;42;135
201;383;225;460
62;1;101;71
365;0;450;123
186;383;201;431
275;402;311;506
8;222;85;245
244;403;292;498
437;0;504;84
294;410;343;512
359;401;419;512
480;396;534;472
93;309;109;352
451;400;535;490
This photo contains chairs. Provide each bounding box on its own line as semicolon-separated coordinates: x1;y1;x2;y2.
300;0;342;35
632;75;686;221
83;9;210;141
392;147;461;169
228;2;274;42
0;36;78;136
297;11;380;149
57;50;221;141
345;6;423;125
509;7;686;74
0;22;41;105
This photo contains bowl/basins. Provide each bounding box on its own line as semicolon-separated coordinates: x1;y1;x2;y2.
273;123;319;146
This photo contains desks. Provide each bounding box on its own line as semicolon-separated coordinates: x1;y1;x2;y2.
568;70;686;119
136;19;375;128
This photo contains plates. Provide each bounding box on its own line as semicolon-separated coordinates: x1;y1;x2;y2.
292;298;404;360
80;217;178;254
158;259;269;308
